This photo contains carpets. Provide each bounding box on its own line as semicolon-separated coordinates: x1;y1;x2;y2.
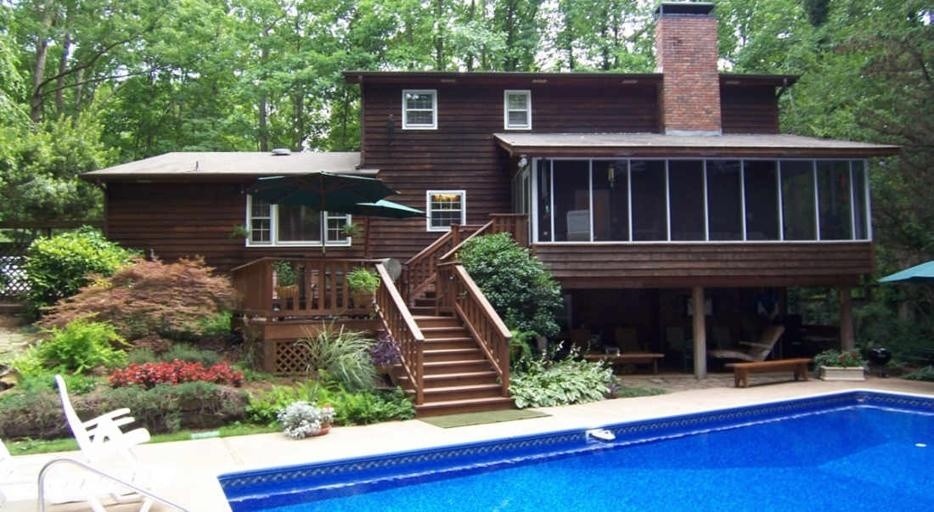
415;408;553;429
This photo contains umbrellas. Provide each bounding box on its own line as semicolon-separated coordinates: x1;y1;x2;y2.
875;260;934;281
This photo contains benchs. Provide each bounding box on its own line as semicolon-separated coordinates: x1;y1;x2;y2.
584;352;665;373
725;358;813;388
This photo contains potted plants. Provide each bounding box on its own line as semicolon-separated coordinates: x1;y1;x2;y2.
343;265;380;307
271;258;297;299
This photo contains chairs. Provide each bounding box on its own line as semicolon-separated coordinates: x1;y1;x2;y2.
57;372;153;512
616;328;642;352
708;324;786;363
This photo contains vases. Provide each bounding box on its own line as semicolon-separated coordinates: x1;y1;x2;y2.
302;404;330;435
820;366;865;382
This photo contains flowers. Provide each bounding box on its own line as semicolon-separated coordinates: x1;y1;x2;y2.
814;346;869;378
277;400;334;439
